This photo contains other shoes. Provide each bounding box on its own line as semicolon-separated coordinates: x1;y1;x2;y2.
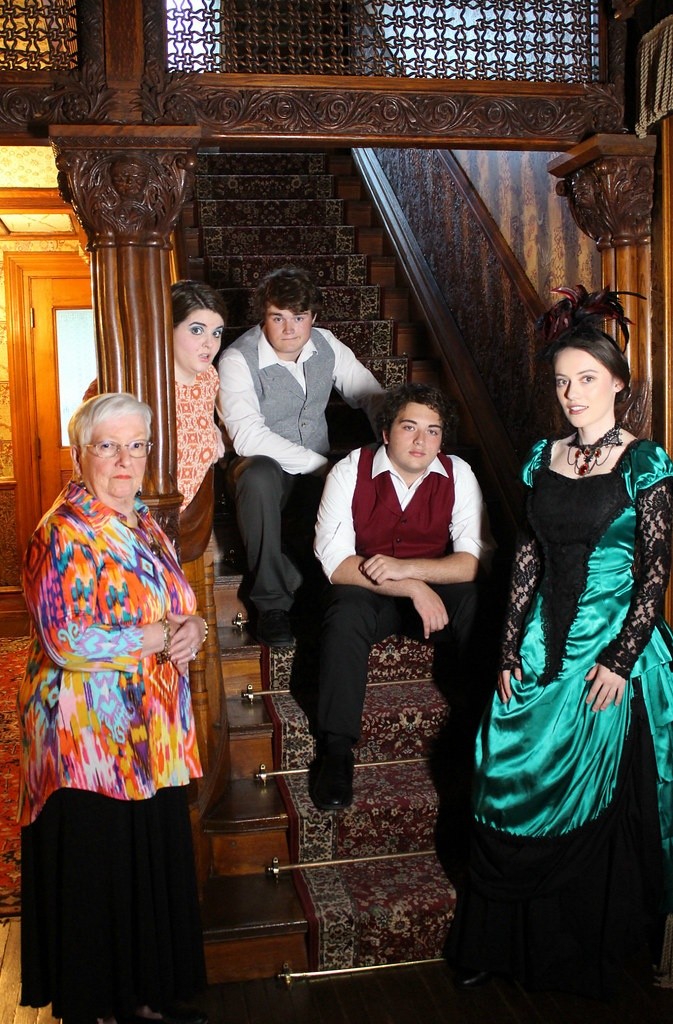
222;544;247;571
119;1007;208;1024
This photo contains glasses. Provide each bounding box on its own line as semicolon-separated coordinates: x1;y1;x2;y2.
85;440;153;458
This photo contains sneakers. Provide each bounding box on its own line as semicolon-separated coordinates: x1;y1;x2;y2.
254;610;294;645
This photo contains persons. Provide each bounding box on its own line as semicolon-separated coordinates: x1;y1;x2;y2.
459;325;673;999
82;279;225;512
216;265;389;646
16;392;209;1024
307;380;504;810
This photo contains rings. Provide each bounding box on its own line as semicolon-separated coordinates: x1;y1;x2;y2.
193;649;199;658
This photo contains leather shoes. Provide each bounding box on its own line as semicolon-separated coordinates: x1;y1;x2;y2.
310;749;354;808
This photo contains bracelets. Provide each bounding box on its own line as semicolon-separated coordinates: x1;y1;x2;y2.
156;618;171;664
201;618;208;642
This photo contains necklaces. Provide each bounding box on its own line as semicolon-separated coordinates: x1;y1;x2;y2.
567;427;621;476
121;520;160;555
